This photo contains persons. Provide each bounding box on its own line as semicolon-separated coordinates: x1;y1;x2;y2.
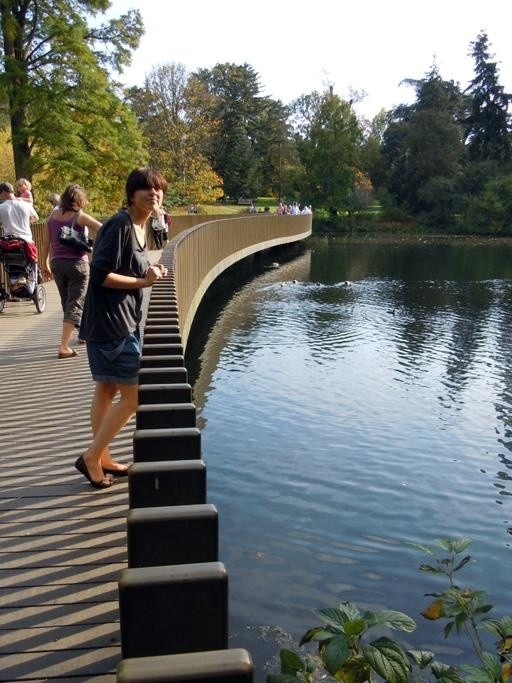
40;184;104;358
150;207;165;251
187;203;195;214
159;208;169;248
47;193;61;217
245;199;313;215
15;177;33;203
0;181;40;303
74;166;169;490
192;205;199;213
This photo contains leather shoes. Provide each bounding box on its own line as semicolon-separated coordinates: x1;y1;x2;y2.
75;455;112;488
103;464;129;478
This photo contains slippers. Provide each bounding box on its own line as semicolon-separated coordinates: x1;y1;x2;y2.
58;348;77;358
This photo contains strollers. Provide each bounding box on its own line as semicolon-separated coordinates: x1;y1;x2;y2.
0;233;48;317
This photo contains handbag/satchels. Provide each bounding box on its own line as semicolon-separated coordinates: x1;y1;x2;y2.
57;228;93;253
